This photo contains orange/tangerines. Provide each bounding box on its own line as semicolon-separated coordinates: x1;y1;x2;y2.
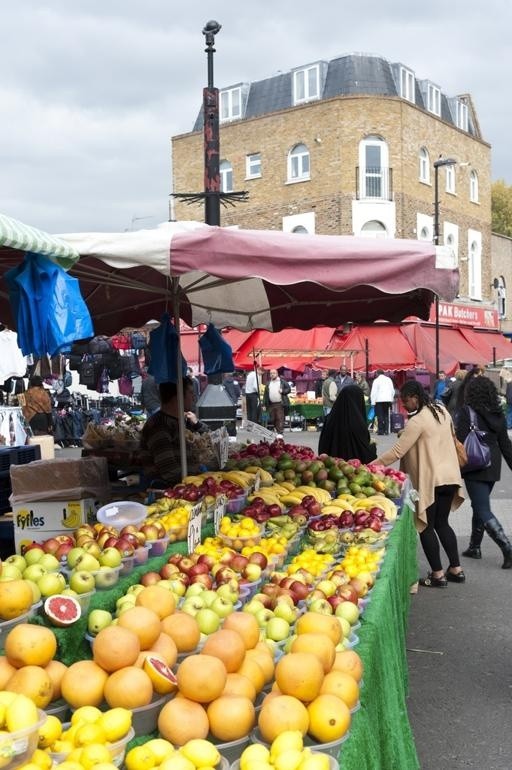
0;580;362;744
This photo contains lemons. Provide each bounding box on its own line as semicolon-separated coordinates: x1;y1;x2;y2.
0;691;328;770
148;506;383;578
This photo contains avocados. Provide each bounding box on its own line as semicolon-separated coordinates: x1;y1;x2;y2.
224;456;399;499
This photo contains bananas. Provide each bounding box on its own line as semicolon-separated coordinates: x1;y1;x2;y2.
182;466;398;521
61;510;81;528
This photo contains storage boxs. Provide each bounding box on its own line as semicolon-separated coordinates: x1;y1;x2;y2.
0;445;41;561
10;456;106;556
391;413;404;432
26;434;55;460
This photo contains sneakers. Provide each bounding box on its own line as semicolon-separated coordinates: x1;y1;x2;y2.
445;567;465;582
419;572;448;587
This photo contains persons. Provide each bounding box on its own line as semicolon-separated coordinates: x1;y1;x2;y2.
140;367;291;489
316;365;511;587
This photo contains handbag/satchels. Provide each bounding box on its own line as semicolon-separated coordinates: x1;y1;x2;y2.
462;430;491;473
453;433;468;468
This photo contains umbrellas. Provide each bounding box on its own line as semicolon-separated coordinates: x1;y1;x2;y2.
51;224;460;484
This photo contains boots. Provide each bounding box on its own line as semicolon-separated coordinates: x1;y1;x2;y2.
483;517;512;568
463;516;483;558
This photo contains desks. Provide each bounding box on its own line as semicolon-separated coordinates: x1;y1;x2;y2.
0;423;430;770
258;400;372;432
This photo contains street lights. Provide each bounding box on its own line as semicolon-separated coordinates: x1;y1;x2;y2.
170;20;249;436
433;152;458;381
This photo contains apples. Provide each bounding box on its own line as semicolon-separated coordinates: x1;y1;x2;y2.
232;439;407;491
159;475;386;533
2;525;374;651
